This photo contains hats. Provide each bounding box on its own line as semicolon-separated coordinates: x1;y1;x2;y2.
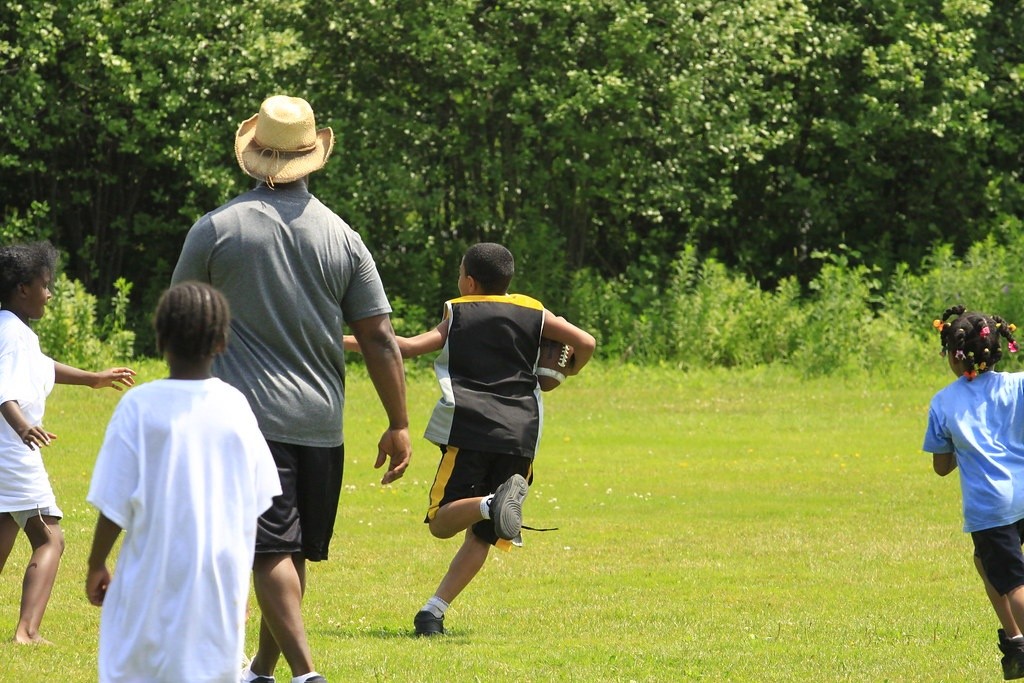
233;95;334;182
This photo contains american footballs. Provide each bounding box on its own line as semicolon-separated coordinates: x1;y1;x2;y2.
536;315;576;392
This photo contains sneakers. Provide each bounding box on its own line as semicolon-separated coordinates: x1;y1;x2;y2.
997;629;1024;679
289;671;326;683
240;654;276;683
490;474;528;540
413;610;444;635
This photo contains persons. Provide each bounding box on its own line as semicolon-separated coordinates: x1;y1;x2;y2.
339;241;599;637
81;277;287;682
923;303;1024;657
0;241;136;651
172;95;414;680
